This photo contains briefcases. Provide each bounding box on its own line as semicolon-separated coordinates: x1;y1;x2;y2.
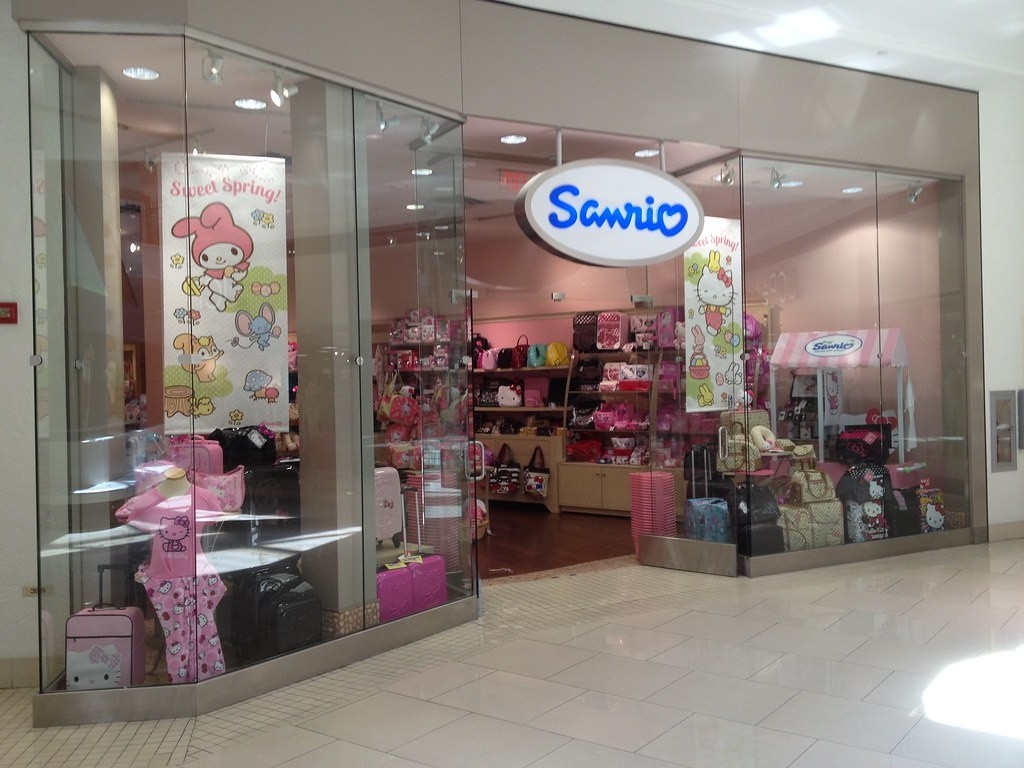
720;410;770;435
572;311;597;352
597;312;628;351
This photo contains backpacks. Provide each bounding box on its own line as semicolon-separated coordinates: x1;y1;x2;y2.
376;372;481;475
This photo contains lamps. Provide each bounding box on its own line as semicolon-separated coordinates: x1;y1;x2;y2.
270;72;299;108
770;167;805;190
375;104;403;133
712;162;735;186
907;183;924;204
418;115;440;145
386;235;398;247
144;152;158;172
201;51;224;85
193;138;211;156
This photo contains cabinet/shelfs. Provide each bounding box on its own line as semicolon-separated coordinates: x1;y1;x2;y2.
378;349;762;521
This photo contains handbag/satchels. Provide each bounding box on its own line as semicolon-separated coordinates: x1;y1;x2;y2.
232;556;323;658
790;469;836;507
523;444;550;498
836;457;893;504
482;348;503;369
527;343;546;367
603;363;626;381
575;360;602;390
473;348;482;369
189;465;245;512
727;481;781;525
241;463;300;547
546;341;570;366
488;443;522;494
512;334;530;368
498;384;523;407
209;422;277;472
738;524;785;557
498;348;512;368
166;434;222;476
572;397;606;429
716;422;762;472
776;439;816;461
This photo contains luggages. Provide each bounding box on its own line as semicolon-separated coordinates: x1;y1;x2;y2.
884;488;920;538
778;505;812;553
687;444;734;501
808;502;844;547
845;496;890;543
375;467;402;540
377;568;412;624
65;564;145;692
399;486;448;614
916;478;945;534
684;445;728;543
